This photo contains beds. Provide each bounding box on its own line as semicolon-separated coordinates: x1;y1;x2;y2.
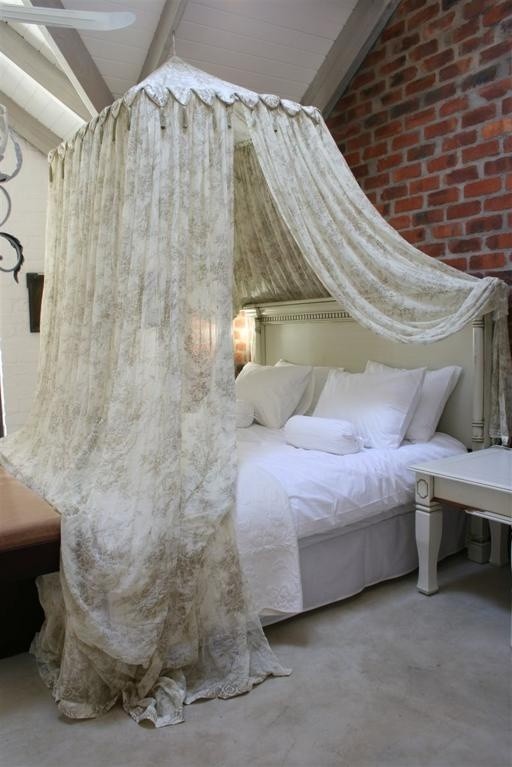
1;278;506;680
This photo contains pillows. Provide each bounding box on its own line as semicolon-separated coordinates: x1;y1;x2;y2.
232;357;463;450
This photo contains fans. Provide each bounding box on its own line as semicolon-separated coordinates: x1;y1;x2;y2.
0;1;136;30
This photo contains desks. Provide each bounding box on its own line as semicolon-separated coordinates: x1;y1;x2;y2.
0;468;63;661
409;445;512;596
